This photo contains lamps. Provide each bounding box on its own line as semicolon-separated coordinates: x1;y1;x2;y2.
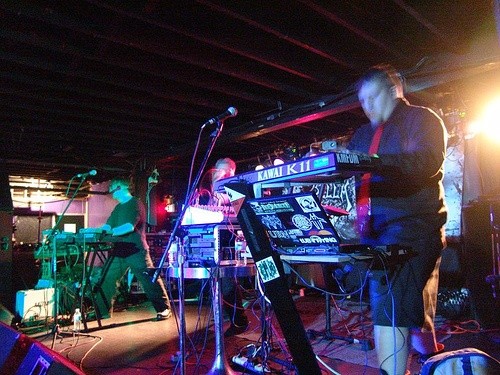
255;153;272;170
273;146;297;166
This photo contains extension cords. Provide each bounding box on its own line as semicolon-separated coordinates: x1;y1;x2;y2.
233;356;268;372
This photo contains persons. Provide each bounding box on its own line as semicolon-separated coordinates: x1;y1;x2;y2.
344;62;448;375
192;157;247;338
86;175;171;321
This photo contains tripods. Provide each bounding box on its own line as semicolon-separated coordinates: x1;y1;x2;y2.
34;176;95;351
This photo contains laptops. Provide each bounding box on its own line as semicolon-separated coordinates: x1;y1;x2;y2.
248;191;375;253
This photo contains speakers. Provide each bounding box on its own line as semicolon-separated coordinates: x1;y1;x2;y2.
0;287;86;375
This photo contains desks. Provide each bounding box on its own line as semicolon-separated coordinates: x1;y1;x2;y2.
241;253;374;344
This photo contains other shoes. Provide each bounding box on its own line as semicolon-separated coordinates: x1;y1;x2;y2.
157;309;172;320
225;320;250;336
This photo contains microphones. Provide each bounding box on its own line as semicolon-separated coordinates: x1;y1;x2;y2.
204;106;238;126
77;170;97;177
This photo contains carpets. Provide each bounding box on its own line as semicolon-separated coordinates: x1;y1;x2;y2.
209;295;454;375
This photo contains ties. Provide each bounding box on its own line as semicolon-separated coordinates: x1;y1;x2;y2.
354;123;386;233
